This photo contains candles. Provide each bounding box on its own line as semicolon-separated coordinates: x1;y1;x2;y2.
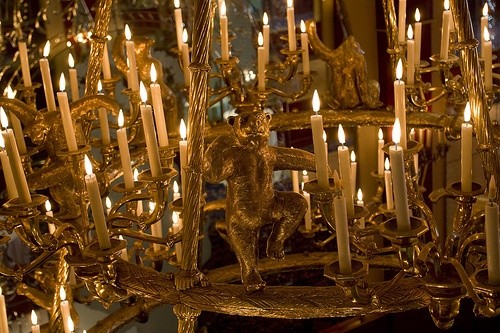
170;179;184;264
171;0;310;93
31;309;41;333
293;57;418;272
483;174;500;286
60;285;75;332
399;1;493;94
178;116;190;208
0;22;168;265
0;286;10;332
460;101;472;189
